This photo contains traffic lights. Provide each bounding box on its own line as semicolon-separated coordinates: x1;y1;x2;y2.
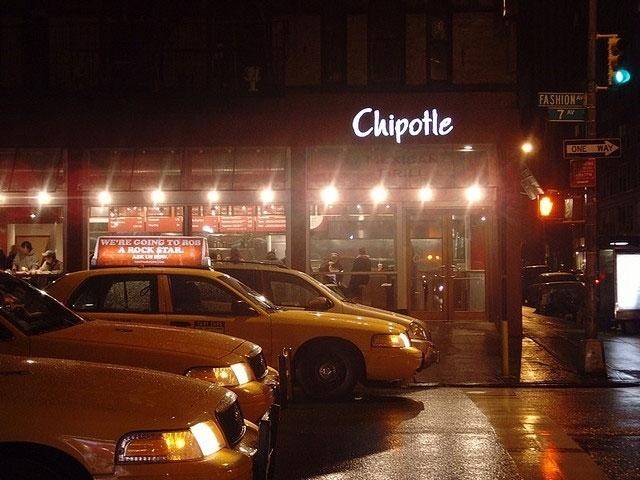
605;34;631;87
538;190;561;222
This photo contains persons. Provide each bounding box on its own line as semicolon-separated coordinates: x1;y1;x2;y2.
208;248;288;265
382;248;397;309
0;240;64;289
317;247;371;303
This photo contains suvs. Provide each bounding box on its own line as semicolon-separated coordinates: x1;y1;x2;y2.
209;259;441;383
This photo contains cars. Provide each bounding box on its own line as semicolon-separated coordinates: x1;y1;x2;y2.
29;234;423;402
0;271;281;427
0;341;264;480
523;262;585;311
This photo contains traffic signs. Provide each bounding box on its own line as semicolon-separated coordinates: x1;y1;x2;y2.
561;137;622;161
535;92;588;123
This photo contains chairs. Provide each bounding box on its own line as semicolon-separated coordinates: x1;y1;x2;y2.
177;281;201;312
